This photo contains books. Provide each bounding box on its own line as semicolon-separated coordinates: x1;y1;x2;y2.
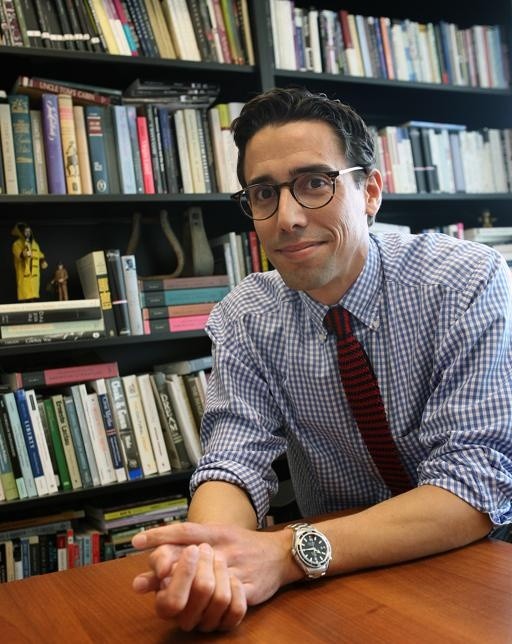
1;491;188;584
267;2;510;87
0;0;255;65
1;355;212;500
368;121;512;194
2;232;268;345
1;76;248;196
371;222;512;268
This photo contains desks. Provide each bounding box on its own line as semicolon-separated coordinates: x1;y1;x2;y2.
0;506;511;644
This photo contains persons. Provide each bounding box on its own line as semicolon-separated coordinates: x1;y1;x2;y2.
12;226;68;302
130;86;512;633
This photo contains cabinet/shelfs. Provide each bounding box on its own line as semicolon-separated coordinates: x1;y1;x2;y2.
0;2;512;580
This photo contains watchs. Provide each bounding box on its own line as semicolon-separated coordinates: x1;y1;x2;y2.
287;520;332;583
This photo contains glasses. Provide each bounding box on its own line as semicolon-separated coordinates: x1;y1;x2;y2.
230;165;364;221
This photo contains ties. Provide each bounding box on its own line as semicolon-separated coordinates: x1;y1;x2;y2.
322;305;414;498
